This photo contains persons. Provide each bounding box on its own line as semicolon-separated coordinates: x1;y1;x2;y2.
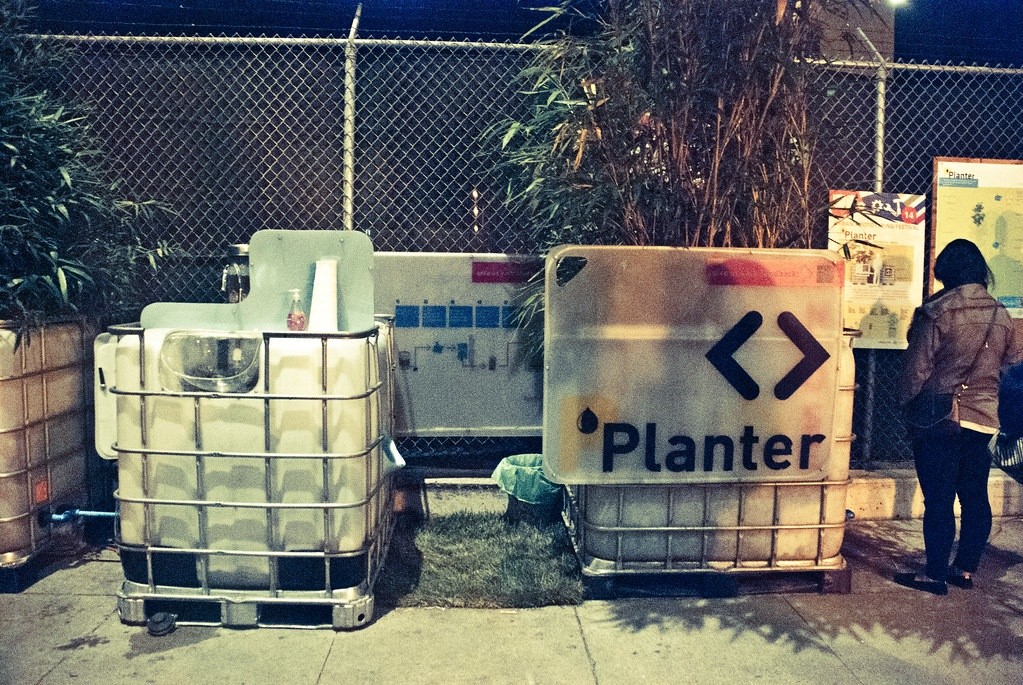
893;239;1017;595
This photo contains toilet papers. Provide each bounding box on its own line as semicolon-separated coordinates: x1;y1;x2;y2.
306;258;339;332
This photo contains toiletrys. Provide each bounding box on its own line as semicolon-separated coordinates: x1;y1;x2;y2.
286;288;306;333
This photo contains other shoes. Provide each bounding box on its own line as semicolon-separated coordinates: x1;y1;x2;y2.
894;572;947;595
945;573;974;588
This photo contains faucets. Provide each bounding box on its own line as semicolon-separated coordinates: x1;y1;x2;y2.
219;243;249;292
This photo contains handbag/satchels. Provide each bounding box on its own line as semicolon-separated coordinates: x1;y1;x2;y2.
904;393;962;439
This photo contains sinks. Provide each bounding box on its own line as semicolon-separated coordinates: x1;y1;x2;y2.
159;328;264;393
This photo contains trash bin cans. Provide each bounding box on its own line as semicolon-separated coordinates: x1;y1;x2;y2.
501;452;562;521
